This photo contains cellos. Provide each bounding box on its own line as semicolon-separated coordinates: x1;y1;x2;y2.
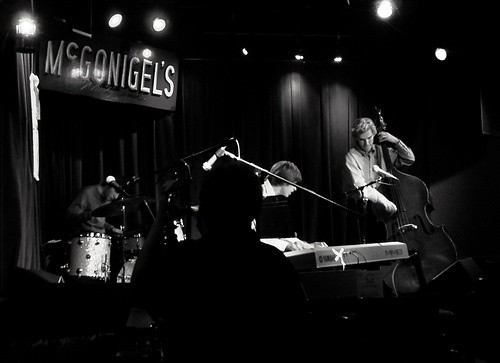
371;106;457;294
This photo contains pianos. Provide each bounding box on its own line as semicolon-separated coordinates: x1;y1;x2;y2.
282;240;410;299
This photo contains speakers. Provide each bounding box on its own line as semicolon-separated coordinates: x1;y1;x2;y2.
296;270;383;298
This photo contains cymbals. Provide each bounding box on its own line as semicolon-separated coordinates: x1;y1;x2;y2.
91;195;141;217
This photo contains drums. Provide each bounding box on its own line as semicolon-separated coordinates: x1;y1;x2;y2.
69;232;112;281
117;256;136;284
120;229;146;253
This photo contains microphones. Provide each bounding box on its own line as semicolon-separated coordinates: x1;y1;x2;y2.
106;176;124;190
202;146;227;171
372;165;399;181
